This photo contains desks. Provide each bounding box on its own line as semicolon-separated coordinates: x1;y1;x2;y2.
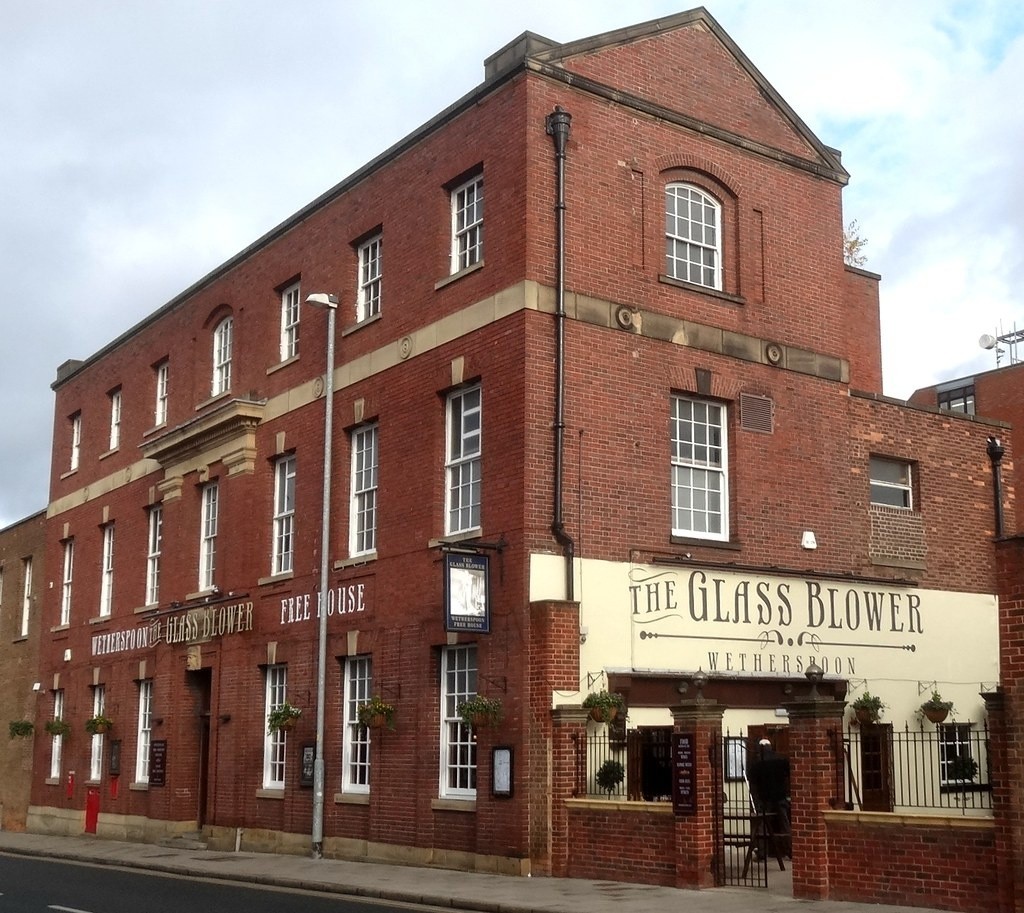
722;812;784;878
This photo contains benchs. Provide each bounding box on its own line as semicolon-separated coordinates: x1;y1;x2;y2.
724;832;791;847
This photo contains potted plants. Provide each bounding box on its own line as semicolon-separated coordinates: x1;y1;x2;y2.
456;694;504;733
267;702;302;736
848;690;885;725
45;715;71;740
579;690;630;733
357;693;395;731
8;718;34;741
85;714;114;734
914;689;960;723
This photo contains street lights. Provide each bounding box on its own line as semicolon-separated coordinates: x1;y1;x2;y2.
307;293;339;862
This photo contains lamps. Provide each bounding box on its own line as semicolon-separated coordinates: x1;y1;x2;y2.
692;666;709;699
804;661;824;700
